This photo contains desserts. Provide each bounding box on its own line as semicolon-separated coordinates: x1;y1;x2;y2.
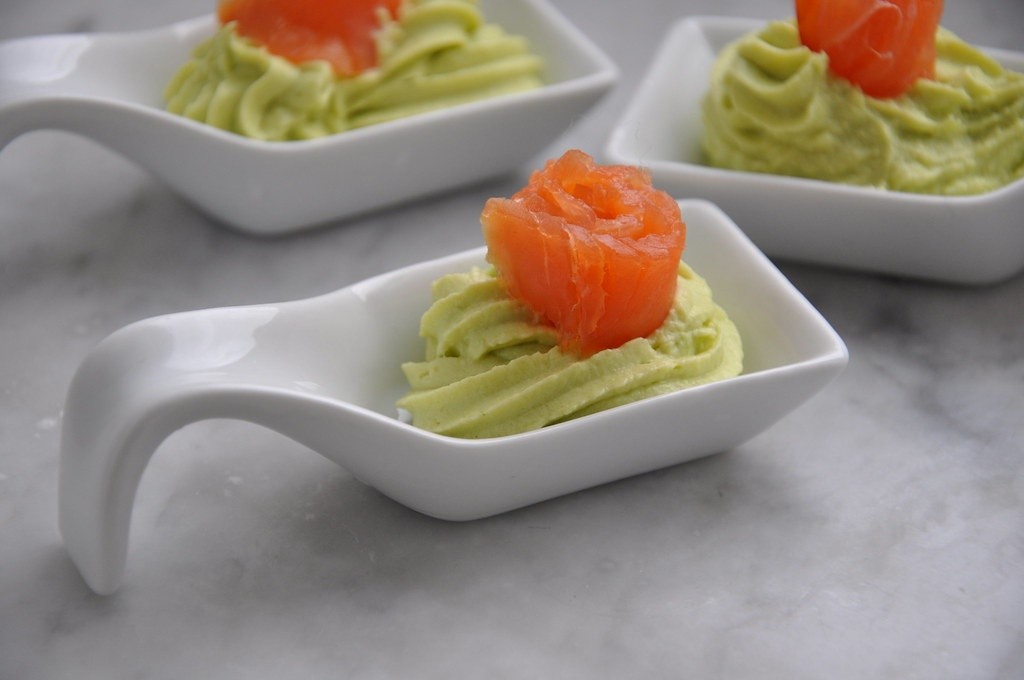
399;153;742;440
696;0;1024;198
159;0;544;143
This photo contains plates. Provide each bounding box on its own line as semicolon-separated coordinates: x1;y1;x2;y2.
2;1;619;240
49;198;851;598
605;12;1023;288
